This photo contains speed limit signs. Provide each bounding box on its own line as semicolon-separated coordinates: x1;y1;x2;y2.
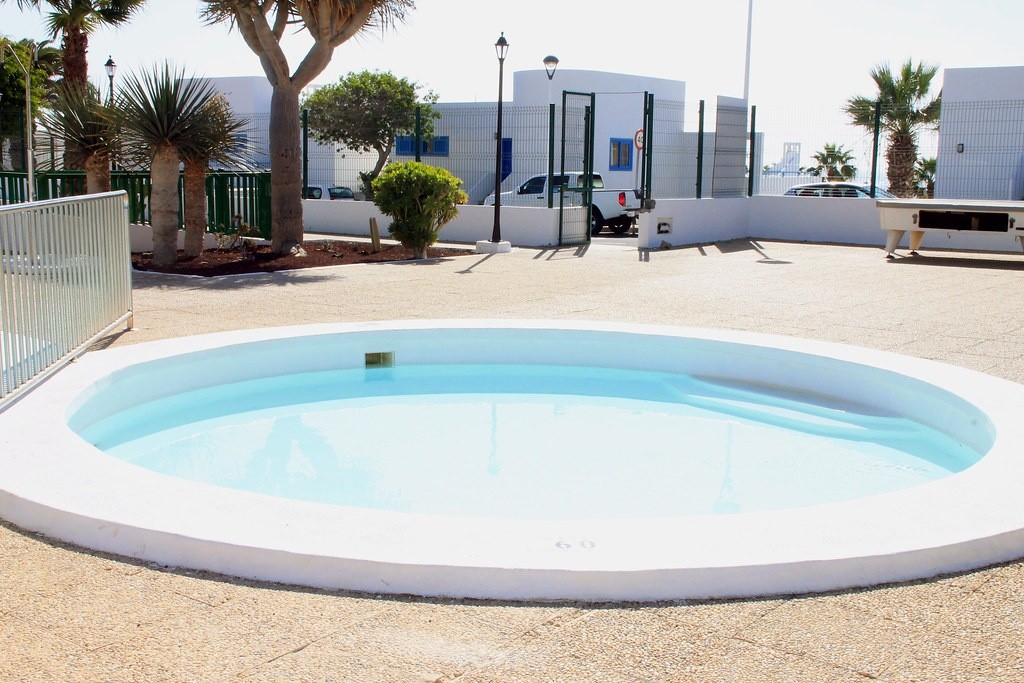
635;129;644;151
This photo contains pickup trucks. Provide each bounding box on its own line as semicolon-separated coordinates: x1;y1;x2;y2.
485;171;642;237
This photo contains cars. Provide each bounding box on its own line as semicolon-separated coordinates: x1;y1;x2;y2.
301;183;356;201
784;181;899;198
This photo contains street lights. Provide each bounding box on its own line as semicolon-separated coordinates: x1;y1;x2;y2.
0;39;55;202
104;54;118;170
489;32;509;242
543;54;560;103
